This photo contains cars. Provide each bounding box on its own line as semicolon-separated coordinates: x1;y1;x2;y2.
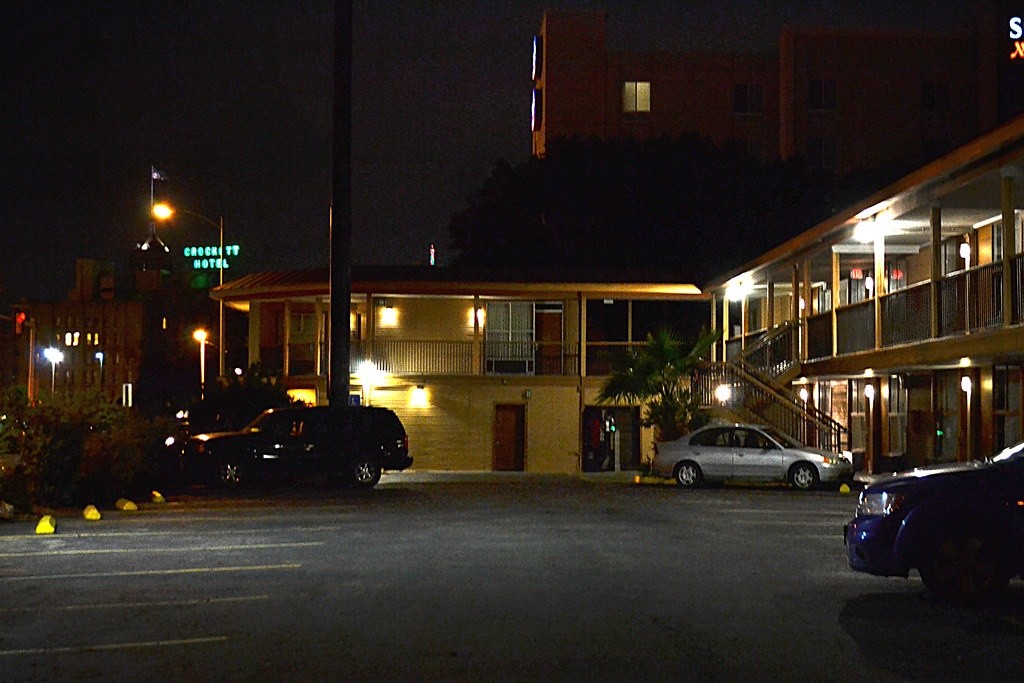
652;421;854;493
845;439;1024;610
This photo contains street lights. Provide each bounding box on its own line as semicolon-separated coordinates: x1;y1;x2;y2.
150;200;223;377
192;327;211;400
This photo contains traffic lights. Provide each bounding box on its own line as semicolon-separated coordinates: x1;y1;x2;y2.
15;311;27;334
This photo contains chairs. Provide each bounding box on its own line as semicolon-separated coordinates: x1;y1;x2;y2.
742;434;761;446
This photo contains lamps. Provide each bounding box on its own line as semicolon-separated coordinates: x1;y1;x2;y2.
522;388;531;399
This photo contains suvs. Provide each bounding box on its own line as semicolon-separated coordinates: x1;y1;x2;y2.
180;403;413;495
